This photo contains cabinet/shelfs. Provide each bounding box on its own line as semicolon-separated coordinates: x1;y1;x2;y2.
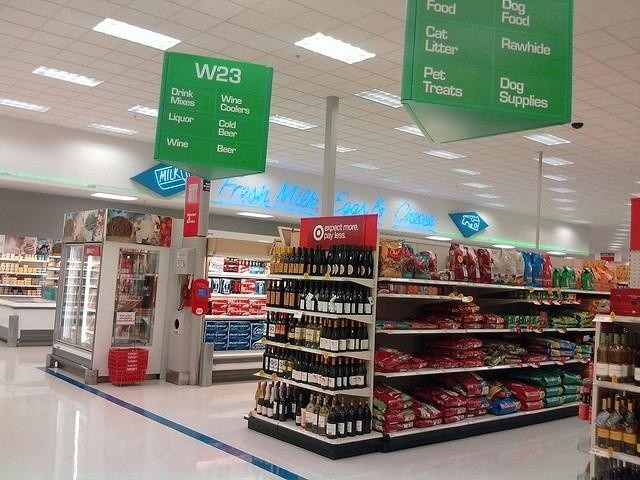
207;271;268;351
374;274;613;438
587;314;640;478
0;253;61;297
254;275;375;445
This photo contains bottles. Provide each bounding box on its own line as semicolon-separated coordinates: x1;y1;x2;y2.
265;311;369;352
594;458;640;480
596;390;640;456
266;280;371;315
263;345;368;391
597;322;640;385
255;381;294;422
270;244;374;279
295;391;372;440
